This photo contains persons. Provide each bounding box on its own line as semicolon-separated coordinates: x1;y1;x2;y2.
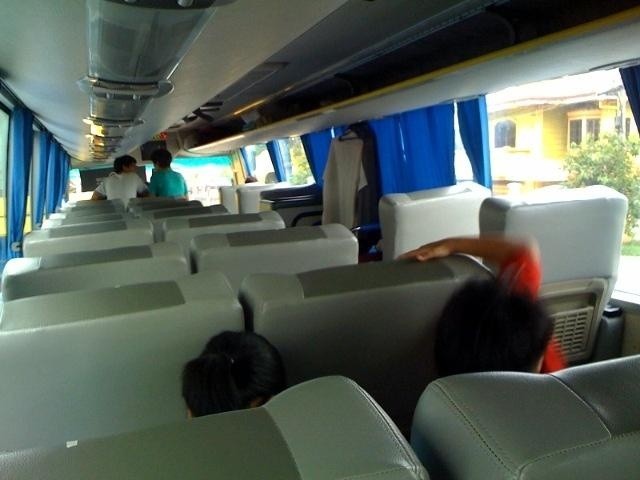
149;149;190;198
178;328;287;420
91;153;149;210
392;230;569;385
244;175;259;183
